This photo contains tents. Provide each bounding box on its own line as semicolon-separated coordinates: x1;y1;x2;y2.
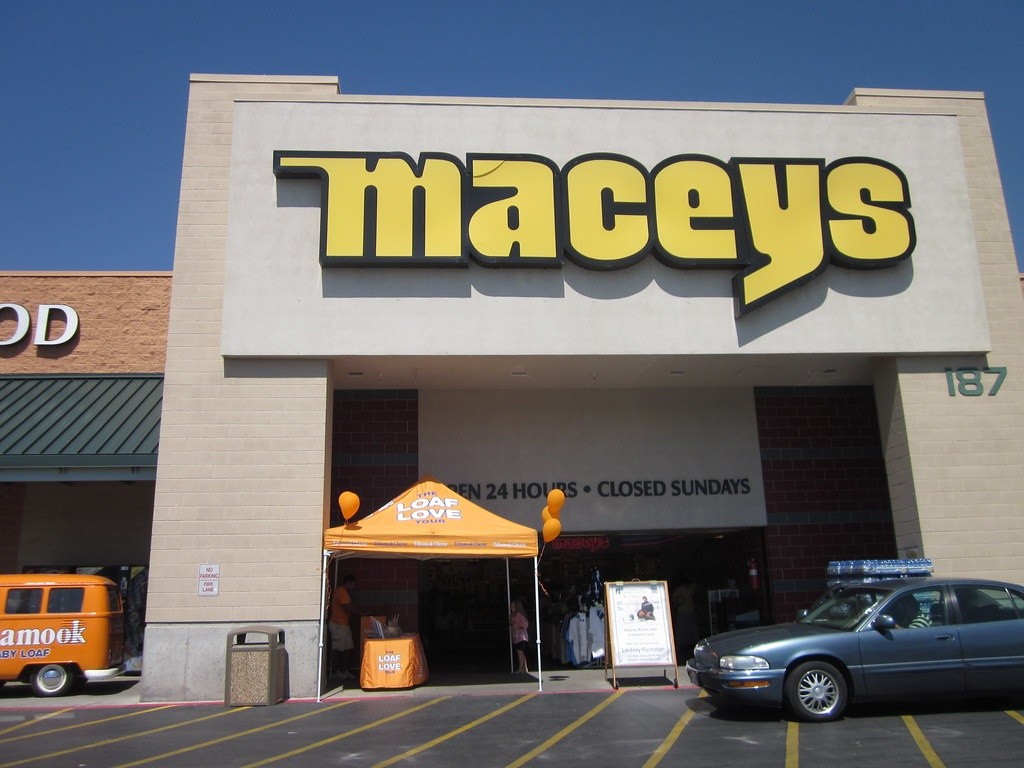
317;480;542;703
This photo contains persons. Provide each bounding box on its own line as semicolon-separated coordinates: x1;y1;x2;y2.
329;575;372;681
671;585;701;653
641;596;654;620
894;594;933;629
509;601;529;675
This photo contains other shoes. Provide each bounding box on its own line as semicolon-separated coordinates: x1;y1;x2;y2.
330;670;347;679
341;669;355;679
515;670;524;675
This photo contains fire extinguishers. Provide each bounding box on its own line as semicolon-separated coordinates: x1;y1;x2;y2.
745;557;762;591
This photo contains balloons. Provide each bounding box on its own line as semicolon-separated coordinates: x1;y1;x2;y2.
542;518;561;542
338;491;351;506
340;493;360;520
547;489;565;514
541;506;560;523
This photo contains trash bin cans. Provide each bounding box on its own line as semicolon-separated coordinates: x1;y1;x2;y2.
225;625;286;708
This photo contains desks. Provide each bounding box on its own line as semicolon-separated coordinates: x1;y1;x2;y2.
359;631;430;690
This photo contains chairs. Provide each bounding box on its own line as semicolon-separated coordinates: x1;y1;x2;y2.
929;602;945;626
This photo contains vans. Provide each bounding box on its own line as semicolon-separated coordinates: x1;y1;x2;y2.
0;574;125;699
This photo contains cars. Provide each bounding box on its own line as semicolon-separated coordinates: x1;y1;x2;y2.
687;575;1024;723
